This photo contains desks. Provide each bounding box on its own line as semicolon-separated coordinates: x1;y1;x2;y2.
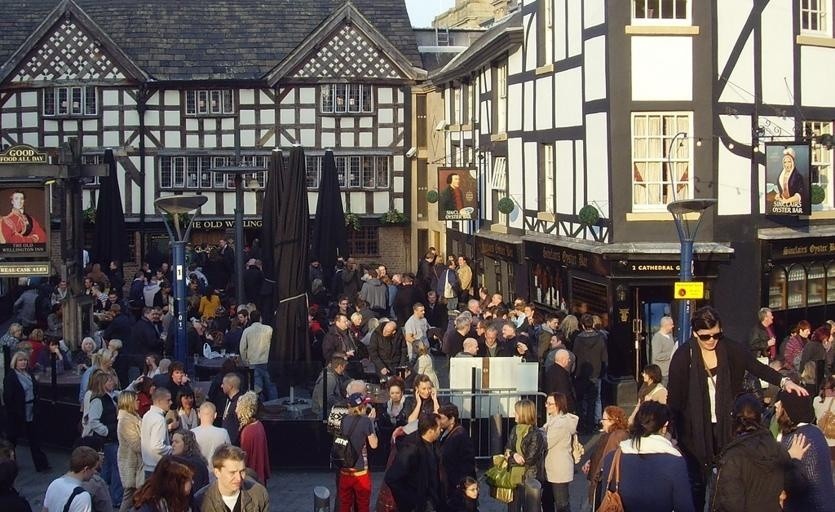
196;354;242;369
265;397;315;421
38;369;82;387
365;382;390;405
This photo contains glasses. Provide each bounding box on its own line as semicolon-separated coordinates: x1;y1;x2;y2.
694;327;724;342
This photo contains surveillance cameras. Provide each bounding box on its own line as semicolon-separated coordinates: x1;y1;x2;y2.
405;146;416;158
435;120;447;132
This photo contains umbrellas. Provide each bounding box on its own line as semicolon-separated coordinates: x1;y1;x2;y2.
264;143;314;412
95;147;126;274
310;148;350;262
261;145;286;309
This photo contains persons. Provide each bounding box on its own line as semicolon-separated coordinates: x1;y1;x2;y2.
0;190;47;244
309;247;609;512
776;150;805;215
587;308;835;512
44;239;279;512
1;277;62;510
442;173;464;216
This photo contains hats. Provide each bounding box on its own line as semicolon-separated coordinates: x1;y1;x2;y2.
347;392;374;407
778;384;819;424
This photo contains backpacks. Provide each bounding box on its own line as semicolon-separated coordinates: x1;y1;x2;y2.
333;414;362;468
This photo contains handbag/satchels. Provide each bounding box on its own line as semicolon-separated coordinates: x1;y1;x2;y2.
596;491;625;512
484;466;518;489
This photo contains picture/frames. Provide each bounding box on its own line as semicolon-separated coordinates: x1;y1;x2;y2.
437;167;477;221
765;141;811;216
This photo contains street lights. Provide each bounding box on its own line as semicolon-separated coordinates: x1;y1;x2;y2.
155;194;210;378
665;199;718;347
210;162;267;315
